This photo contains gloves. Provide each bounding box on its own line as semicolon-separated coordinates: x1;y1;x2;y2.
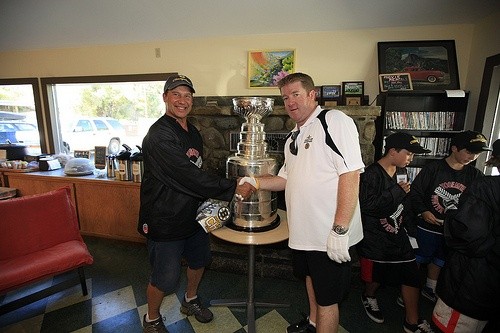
235;176;258;201
327;230;351;264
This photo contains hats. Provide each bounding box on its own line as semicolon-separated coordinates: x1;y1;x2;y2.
384;132;430;153
455;132;493;152
164;76;196;94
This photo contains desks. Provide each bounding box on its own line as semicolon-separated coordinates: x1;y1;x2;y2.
210;208;290;333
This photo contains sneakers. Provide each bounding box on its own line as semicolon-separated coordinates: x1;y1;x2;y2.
398;296;406;308
404;320;434;333
285;317;316;333
423;288;439;303
143;313;169;333
360;292;385;323
180;292;213;323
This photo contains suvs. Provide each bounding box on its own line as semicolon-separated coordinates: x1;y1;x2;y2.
60;117;126;156
0;111;41;144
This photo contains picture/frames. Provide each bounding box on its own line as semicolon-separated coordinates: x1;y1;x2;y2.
379;73;413;92
315;81;364;106
378;39;460;93
248;49;296;89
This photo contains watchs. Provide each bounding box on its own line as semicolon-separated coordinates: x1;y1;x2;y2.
332;223;349;235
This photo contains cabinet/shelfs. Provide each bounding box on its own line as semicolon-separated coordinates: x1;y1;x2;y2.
5;170;147;242
376;89;471;186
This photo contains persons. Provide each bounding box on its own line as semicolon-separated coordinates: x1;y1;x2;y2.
360;130;500;333
137;75;257;333
235;73;365;333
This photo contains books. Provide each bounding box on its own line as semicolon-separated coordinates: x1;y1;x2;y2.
382;111;455;184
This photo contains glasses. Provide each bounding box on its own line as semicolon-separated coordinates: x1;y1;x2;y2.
289;130;300;156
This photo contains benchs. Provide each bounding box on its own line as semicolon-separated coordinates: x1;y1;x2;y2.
0;186;94;316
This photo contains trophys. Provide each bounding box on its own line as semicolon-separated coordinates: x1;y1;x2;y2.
223;96;282;233
